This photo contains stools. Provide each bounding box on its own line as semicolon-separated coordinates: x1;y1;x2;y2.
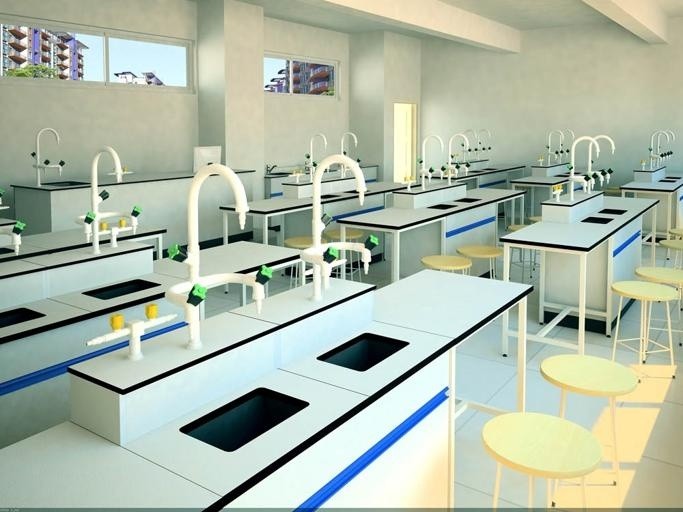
283;229;365;289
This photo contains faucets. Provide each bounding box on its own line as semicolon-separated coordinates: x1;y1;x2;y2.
304;132;361;177
12;219;26;257
650;129;675;162
419;134;471;183
301;156;380;290
548;130;573;160
460;130;492;157
165;162;272;343
35;129;65;177
571;134;615;196
76;147;142;243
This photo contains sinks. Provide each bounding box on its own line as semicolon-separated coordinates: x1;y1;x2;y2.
316;334;406;372
180;387;310;454
